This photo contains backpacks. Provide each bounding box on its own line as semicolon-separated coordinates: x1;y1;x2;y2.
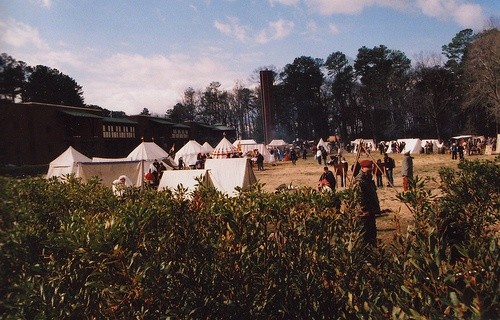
384;157;395;168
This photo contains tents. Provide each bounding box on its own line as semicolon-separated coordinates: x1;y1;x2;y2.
377;138;413;153
158;157;256;204
211;136;271;159
448;135;472;147
46;142;174;191
401;138;422;155
421;139;439;148
267;139;285;146
174;139;213;168
317;138;375;152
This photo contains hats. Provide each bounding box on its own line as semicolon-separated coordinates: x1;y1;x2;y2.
360;160;373;168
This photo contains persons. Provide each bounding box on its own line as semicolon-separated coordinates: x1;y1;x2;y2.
111;153;212;198
376;153;395;187
402;150;413;192
450;136;497;159
272;142;372;194
379;142;388;155
225;149;265;172
353;160;380;248
390;141;406;154
425;140;433;154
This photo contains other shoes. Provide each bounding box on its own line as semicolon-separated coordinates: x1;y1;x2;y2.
387;184;394;187
